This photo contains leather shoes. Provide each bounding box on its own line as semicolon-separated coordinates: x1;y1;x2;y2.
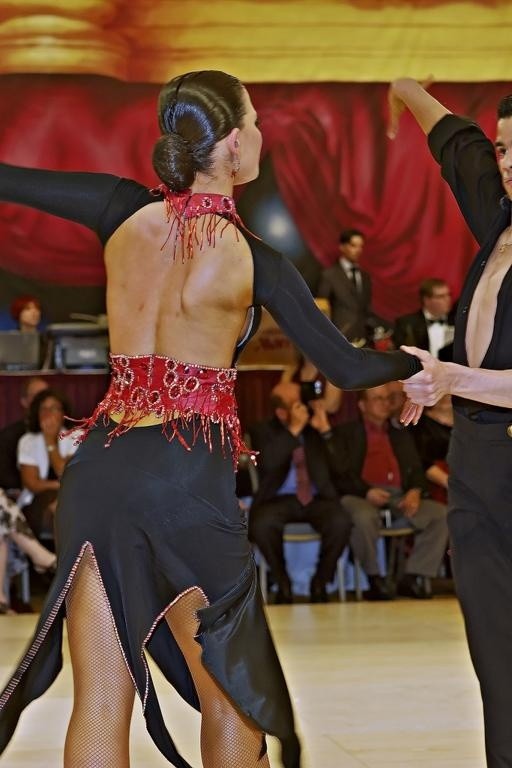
274;578;434;604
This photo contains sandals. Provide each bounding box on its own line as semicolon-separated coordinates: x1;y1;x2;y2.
0;599;12;614
34;557;60;575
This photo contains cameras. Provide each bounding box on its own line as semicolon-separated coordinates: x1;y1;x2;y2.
300;379;323;416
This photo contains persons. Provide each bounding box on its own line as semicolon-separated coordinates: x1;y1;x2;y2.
0;377;85;615
9;292;58;370
311;229;372;343
0;68;429;768
386;74;512;767
238;332;464;602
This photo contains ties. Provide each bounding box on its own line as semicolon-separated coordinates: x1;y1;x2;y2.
293;441;313;507
350;265;358;292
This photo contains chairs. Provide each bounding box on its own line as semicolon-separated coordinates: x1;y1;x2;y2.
238;466;436;601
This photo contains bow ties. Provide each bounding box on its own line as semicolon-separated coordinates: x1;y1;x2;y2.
426;317;445;327
